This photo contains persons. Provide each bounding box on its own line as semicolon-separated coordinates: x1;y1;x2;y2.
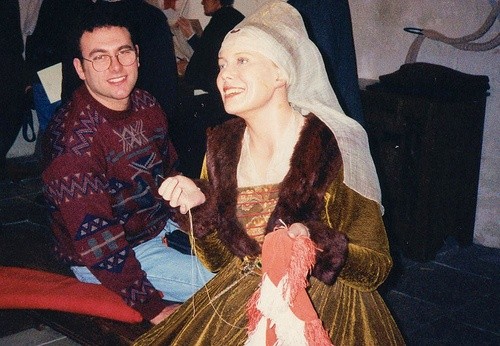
131;0;403;344
35;13;220;329
177;0;247;128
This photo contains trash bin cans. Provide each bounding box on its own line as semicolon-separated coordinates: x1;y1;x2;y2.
369;61;492;265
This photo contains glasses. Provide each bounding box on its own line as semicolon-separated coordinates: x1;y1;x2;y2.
82;50;137;72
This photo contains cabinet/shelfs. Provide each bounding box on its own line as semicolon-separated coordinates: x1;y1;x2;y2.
359;61;491;263
